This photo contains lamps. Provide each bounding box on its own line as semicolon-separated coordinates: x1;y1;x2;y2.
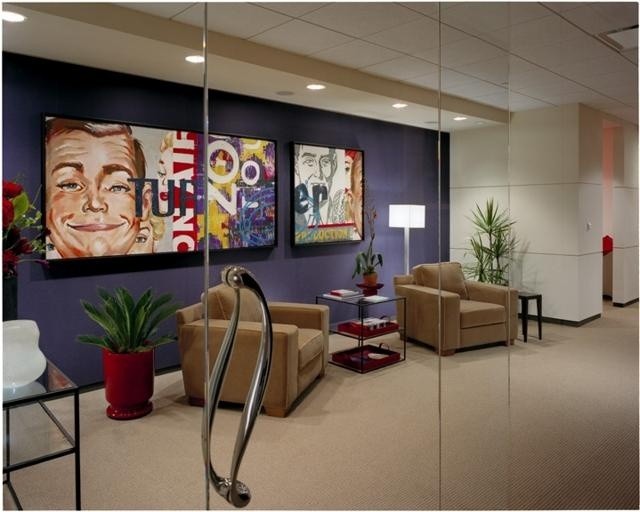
387;203;426;277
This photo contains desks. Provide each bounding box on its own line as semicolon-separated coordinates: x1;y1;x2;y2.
2;359;82;511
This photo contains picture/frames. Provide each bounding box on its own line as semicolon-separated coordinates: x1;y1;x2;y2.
42;112;279;265
289;139;365;247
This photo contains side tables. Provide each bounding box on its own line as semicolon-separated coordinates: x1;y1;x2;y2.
517;291;543;344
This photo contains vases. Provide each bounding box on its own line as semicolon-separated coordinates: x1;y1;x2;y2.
2;274;19;321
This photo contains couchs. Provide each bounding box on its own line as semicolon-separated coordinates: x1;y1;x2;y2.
175;278;330;419
393;260;519;356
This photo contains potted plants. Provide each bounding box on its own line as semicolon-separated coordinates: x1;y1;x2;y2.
350;207;384;297
77;284;186;421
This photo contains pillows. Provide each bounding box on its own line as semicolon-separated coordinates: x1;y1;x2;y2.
410;261;470;300
199;282;264;323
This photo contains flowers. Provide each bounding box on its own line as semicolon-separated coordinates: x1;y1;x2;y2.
1;179;55;273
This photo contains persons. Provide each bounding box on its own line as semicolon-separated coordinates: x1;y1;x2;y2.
44;117;154;257
346;153;365;238
128;185;163;255
328;149;354;226
155;131;188;217
293;145;337;208
